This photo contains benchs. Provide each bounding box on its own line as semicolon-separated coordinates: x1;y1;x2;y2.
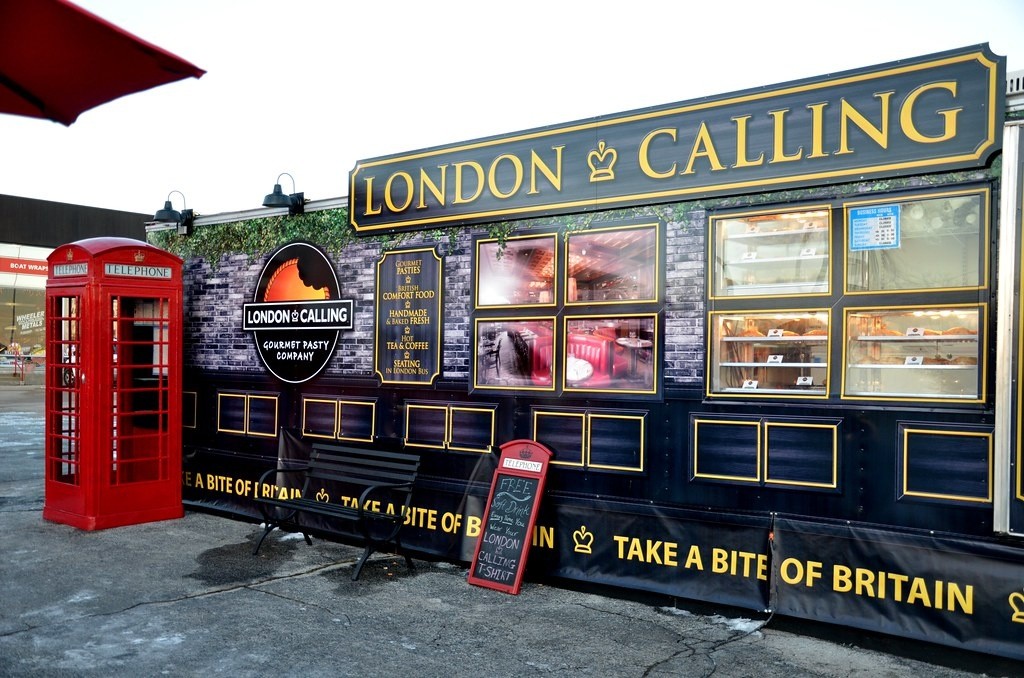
252;443;423;582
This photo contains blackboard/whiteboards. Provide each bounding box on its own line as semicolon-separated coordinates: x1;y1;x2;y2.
468;468;545;596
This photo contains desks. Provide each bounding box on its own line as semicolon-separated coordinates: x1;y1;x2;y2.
0;353;70;385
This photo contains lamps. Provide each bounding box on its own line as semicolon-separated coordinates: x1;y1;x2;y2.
152;191;194;236
262;173;305;217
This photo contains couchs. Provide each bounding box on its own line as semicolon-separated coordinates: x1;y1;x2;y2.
566;315;629;392
533;336;553;387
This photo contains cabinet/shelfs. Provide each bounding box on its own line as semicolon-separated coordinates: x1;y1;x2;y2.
711;311;830;398
843;307;980;401
714;206;830;296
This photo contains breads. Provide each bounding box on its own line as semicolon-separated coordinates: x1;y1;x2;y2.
734;328;828;337
854;326;977;365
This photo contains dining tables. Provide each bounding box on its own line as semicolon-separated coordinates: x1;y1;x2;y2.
518;325;540;348
480;339;496;347
616;337;653;383
478;346;494;383
566;357;593;387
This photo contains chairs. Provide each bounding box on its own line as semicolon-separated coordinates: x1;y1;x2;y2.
478;321;539;384
